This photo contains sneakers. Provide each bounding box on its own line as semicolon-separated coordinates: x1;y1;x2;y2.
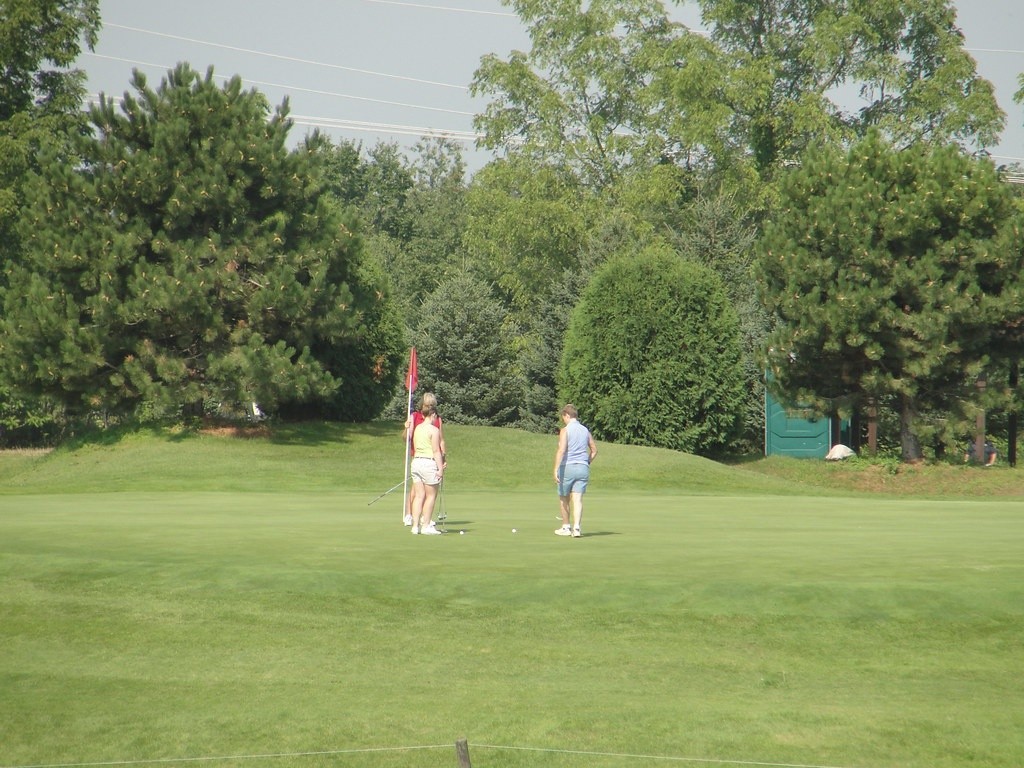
572;529;581;537
555;527;572;535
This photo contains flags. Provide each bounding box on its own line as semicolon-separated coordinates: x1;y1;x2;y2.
405;348;418;392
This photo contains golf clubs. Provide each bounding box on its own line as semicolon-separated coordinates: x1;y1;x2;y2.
437;476;446;520
440;475;447;533
367;477;413;506
555;493;573;521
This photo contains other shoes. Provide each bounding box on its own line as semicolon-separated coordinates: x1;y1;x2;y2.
418;515;436;526
410;527;419;534
404;515;413;526
420;525;441;535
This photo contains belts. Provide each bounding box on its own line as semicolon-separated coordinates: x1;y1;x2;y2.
414;456;436;461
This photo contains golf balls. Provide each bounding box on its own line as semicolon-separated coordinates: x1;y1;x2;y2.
460;530;464;535
512;529;517;533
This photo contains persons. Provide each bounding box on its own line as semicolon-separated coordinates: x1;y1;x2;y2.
403;392;446;535
554;404;598;537
964;436;997;466
